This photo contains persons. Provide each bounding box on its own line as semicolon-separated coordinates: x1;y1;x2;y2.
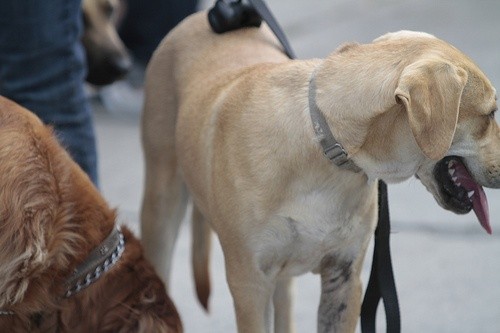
0;0;97;188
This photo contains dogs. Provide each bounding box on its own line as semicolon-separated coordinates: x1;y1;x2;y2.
138;10;500;333
0;96;185;333
